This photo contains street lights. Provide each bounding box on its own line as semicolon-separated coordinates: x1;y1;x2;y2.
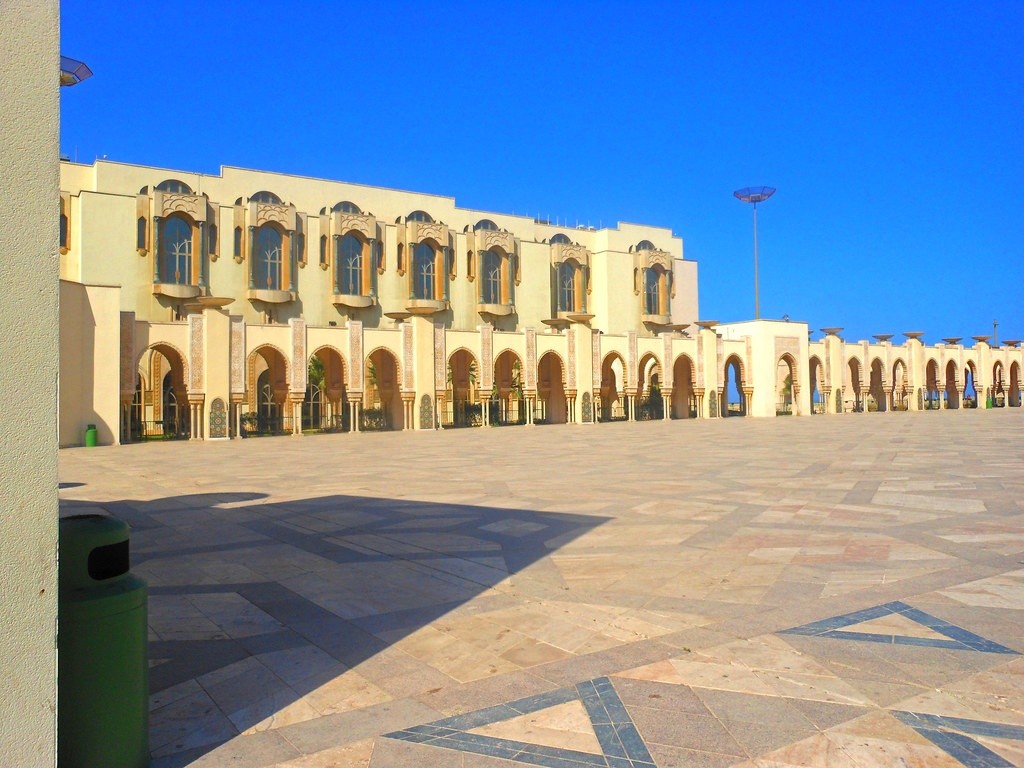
733;184;777;320
993;318;999;346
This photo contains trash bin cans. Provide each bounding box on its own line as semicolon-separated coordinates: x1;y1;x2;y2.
986;398;992;409
85;424;97;448
57;514;147;768
